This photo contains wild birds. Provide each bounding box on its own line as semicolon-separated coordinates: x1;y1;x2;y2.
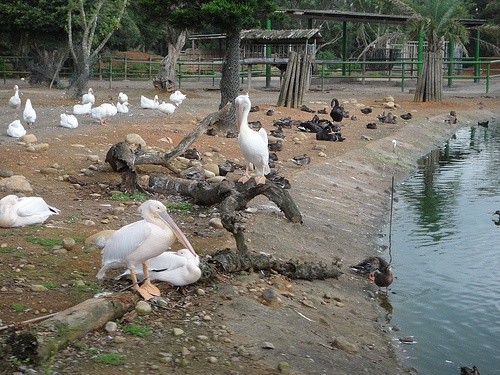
368;257;394;296
460;366;479;375
8;84;21;111
235;96;271;186
22;97;36;126
59;112;79;129
493;210;500;217
140;89;186;115
0;194;62;229
7;119;27;138
73;87;130;126
248;106;457;190
96;199;202;302
331;98;344;123
